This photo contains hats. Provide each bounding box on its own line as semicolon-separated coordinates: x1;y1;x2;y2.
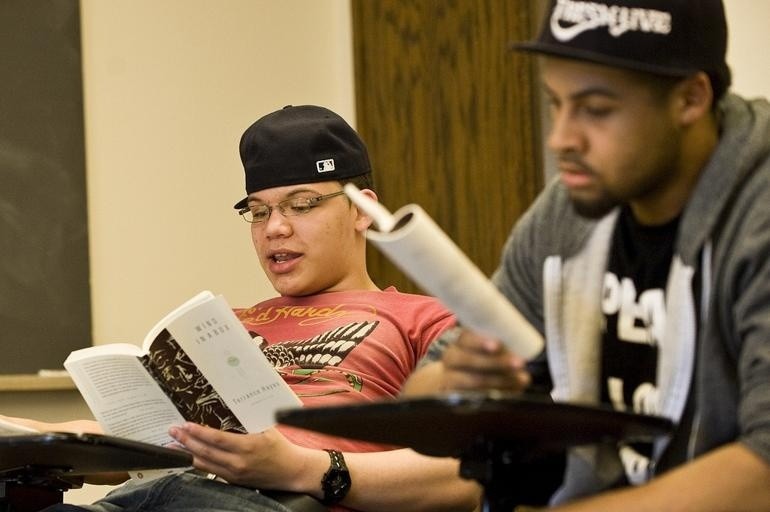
511;0;729;79
234;105;371;209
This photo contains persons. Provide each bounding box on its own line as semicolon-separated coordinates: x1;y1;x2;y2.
399;1;770;512
38;104;485;512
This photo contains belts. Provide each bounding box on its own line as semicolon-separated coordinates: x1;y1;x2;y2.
183;459;322;510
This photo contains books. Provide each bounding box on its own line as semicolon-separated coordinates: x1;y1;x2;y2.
63;291;300;453
342;180;547;363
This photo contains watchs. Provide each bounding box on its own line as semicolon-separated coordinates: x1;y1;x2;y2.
315;449;351;506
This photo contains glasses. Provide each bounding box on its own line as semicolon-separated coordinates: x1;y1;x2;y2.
238;189;345;224
